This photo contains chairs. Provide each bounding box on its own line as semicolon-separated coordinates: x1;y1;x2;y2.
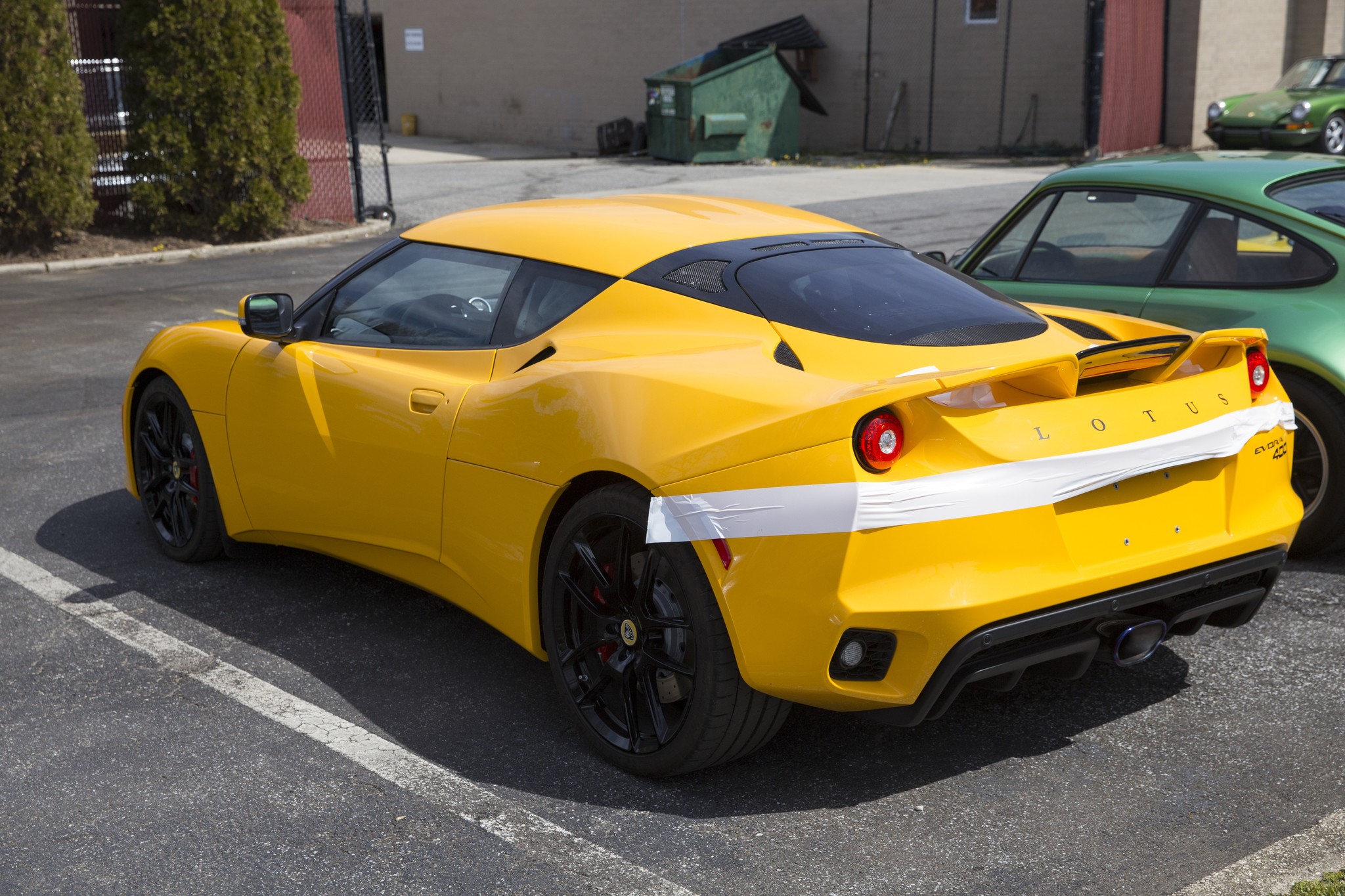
1288;205;1345;281
1186;217;1241;283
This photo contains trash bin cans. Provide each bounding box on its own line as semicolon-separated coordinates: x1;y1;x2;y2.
641;41;830;165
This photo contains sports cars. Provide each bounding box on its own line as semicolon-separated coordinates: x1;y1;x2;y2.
918;151;1345;553
1205;55;1345;155
117;194;1304;778
